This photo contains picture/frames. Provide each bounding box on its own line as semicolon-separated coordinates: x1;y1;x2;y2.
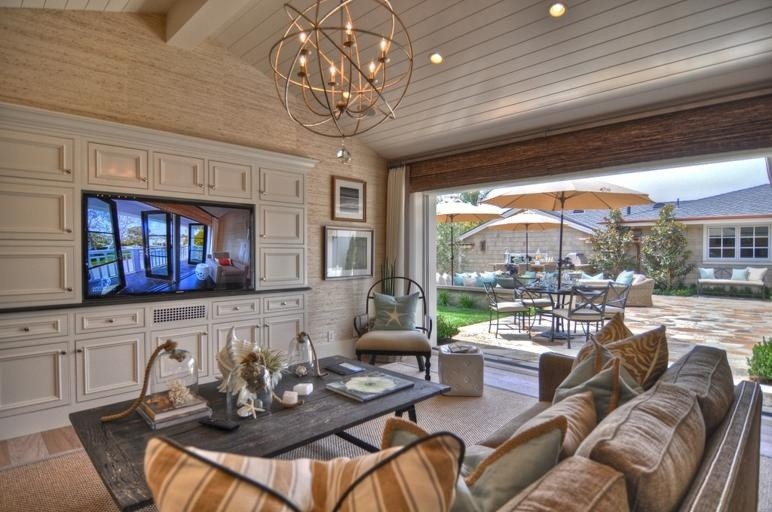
324;225;375;280
331;175;366;222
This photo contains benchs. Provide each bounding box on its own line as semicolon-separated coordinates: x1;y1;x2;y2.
697;266;766;300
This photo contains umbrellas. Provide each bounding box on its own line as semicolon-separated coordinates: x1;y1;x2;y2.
436;199;502;286
486;211;568;272
478;180;656;332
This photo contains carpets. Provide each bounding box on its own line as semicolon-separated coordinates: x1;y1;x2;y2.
0;363;539;511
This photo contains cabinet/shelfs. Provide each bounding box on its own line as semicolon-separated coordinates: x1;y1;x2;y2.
0;102;321;438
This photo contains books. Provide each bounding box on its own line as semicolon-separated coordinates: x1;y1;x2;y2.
325;371;414;402
135;395;213;431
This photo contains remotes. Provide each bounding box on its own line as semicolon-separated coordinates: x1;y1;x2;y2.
197;416;240;431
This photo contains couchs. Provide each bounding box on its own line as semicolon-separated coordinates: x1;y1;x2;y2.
460;352;764;512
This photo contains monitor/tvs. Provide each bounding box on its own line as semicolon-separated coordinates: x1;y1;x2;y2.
81;189;255;305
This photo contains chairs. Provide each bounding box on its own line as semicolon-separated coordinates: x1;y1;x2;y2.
606;273;655;307
484;282;631;352
354;276;432;382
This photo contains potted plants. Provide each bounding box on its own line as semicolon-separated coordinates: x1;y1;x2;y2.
747;336;772;413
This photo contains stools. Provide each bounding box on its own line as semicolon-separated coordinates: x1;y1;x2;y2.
438;343;483;397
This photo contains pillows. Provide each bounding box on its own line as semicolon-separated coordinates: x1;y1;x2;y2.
436;268;634;294
511;392;598;456
141;431;466;511
382;414;568;512
498;454;631;512
698;266;768;281
574;380;708;512
660;346;736;435
548;311;668;423
372;291;419;330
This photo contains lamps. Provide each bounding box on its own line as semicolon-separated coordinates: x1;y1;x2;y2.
269;0;414;166
